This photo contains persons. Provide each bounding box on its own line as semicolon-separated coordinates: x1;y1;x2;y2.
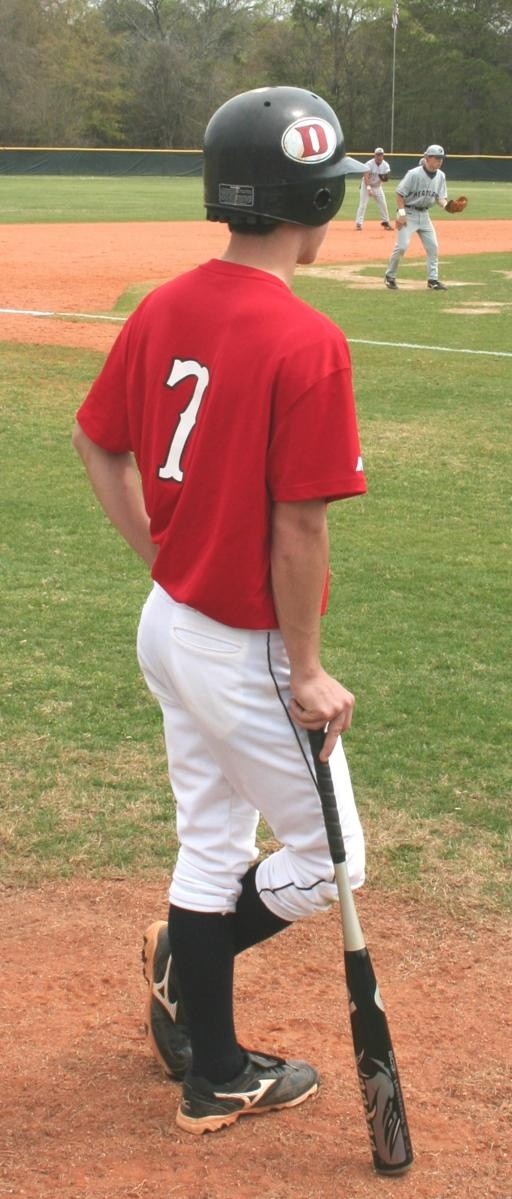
354;147;395;232
69;84;372;1139
384;143;469;291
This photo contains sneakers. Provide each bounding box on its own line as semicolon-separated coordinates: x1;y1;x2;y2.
356;222;393;231
177;1042;317;1135
140;920;194;1084
427;280;448;291
383;276;398;289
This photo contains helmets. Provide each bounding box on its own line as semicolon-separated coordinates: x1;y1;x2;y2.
419;144;445;165
203;86;370;229
374;148;384;154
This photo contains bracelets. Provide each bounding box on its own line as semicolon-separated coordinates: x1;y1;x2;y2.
397;208;406;218
367;185;372;190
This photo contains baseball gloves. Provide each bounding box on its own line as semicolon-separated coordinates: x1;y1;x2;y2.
445;196;468;213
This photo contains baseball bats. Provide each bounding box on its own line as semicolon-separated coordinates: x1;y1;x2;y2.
309;729;414;1176
